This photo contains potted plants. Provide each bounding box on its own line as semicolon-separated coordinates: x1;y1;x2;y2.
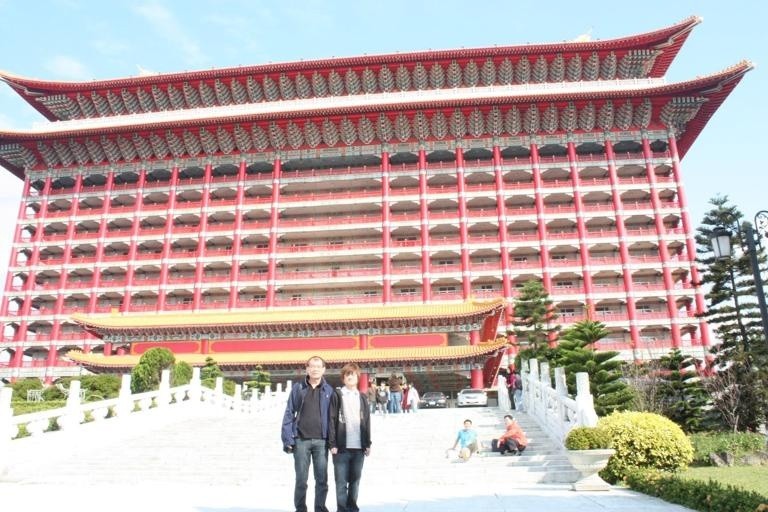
564;425;616;491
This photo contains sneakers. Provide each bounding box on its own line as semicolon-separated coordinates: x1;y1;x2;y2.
514;450;521;456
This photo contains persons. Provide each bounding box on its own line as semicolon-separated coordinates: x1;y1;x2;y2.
280;355;334;512
328;362;372;512
496;414;529;456
452;418;480;461
504;364;516;410
515;372;523;411
368;374;420;414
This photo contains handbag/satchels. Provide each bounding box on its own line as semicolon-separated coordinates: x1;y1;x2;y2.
284;421;298;453
491;439;504;452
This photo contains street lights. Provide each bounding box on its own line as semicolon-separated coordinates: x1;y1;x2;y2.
709;210;768;345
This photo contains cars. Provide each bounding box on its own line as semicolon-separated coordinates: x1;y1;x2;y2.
455;385;488;406
418;391;450;408
